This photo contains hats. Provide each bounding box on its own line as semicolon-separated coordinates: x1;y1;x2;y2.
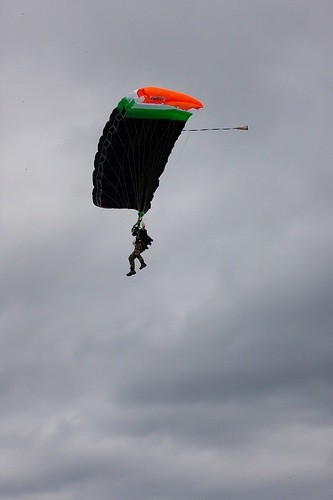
132;226;138;236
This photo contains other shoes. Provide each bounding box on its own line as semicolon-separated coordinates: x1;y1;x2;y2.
139;263;147;269
127;270;137;276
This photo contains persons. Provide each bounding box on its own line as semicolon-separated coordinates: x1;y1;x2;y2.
125;219;148;277
